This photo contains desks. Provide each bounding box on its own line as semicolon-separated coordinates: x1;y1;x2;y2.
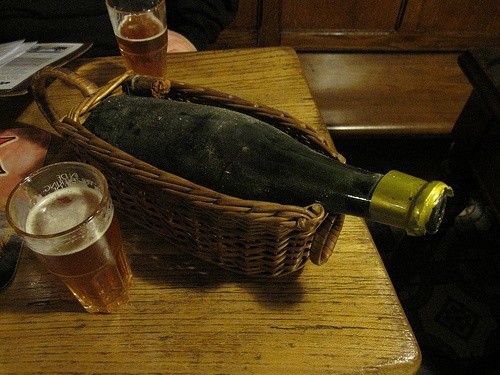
0;45;422;374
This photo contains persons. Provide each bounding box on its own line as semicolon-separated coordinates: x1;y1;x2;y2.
0;0;240;58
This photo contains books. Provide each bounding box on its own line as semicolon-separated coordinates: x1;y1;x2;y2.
0;39;93;96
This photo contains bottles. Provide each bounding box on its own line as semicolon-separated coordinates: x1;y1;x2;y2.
83;93;453;237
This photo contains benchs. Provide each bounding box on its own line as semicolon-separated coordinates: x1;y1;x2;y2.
295;46;500;138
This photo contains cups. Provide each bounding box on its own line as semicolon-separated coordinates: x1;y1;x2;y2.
5;161;133;314
105;0;168;78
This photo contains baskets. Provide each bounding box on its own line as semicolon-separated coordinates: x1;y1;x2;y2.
30;63;345;284
